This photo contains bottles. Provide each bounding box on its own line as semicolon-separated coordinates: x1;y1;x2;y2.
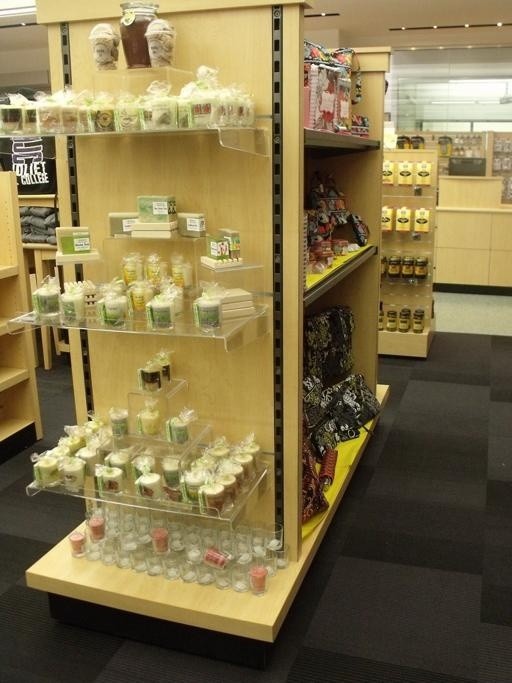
120;2;159;68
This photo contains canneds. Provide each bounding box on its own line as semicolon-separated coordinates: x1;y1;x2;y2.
380;307;425;334
382;255;428;278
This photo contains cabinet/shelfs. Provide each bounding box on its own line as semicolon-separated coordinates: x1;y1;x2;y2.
378;149;439;358
0;171;47;441
1;105;72;369
302;132;391;549
399;132;512;207
10;2;297;641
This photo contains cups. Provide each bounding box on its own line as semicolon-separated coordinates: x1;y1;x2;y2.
68;499;289;596
35;86;254;134
138;367;163;392
157;361;172;384
144;18;177;68
37;260;223;331
88;23;119;71
33;408;264;516
